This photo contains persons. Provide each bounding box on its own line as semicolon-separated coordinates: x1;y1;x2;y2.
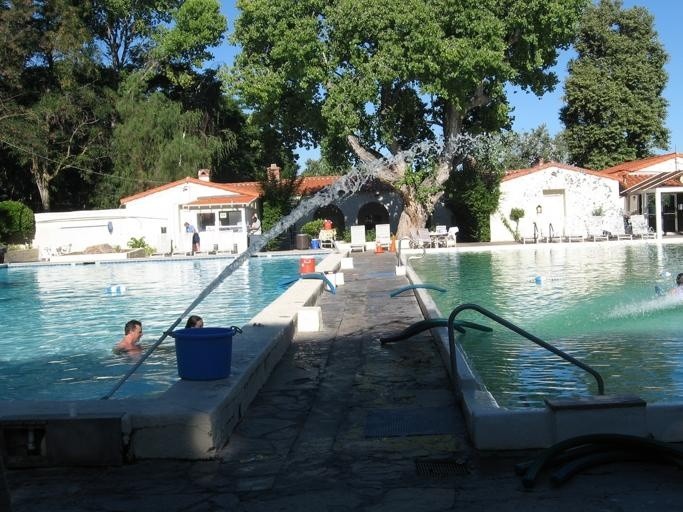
185;315;203;328
112;319;147;353
666;273;683;295
249;212;262;235
183;221;200;254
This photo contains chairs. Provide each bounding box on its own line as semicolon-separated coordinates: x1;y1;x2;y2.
408;225;459;249
562;214;656;243
349;223;392;254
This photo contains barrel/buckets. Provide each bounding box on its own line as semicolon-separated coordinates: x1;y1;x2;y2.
299;254;316;273
162;325;243;382
310;239;321;249
323;219;332;229
295;233;309;250
